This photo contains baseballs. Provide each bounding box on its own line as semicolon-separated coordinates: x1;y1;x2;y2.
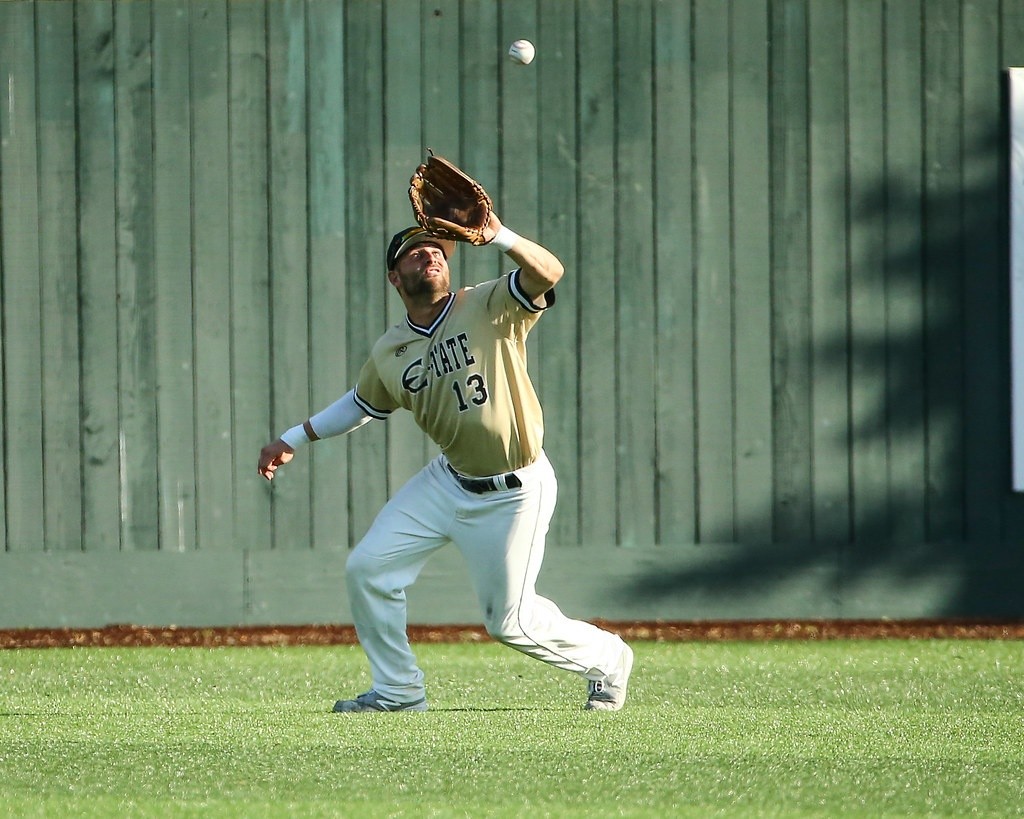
509;39;535;65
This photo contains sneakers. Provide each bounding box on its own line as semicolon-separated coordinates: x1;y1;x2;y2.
332;689;427;713
584;642;633;711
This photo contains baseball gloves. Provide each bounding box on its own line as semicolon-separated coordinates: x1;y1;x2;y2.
407;155;492;246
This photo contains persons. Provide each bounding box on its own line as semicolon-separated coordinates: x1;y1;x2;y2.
257;156;634;713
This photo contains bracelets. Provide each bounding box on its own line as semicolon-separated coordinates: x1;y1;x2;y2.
490;225;517;253
280;424;311;450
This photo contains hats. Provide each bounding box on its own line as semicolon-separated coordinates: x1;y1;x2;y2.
386;227;457;272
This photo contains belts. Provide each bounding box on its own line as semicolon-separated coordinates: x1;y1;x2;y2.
446;463;522;495
